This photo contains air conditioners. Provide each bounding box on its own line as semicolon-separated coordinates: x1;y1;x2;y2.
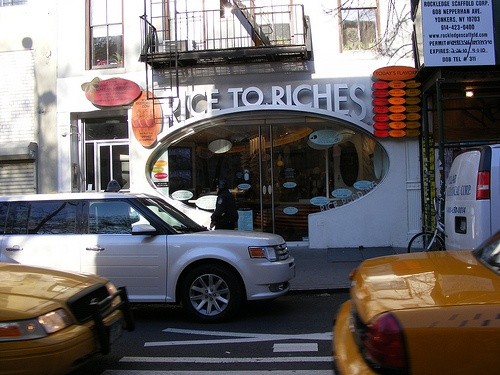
165;39;188;53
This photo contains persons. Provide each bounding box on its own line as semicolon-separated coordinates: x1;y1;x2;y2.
209;179;239;230
104;179;121;193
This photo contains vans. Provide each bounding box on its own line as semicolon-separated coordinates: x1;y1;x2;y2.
439;144;500;253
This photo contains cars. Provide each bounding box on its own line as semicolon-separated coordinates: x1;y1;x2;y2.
0;191;295;323
0;263;135;375
329;229;499;375
